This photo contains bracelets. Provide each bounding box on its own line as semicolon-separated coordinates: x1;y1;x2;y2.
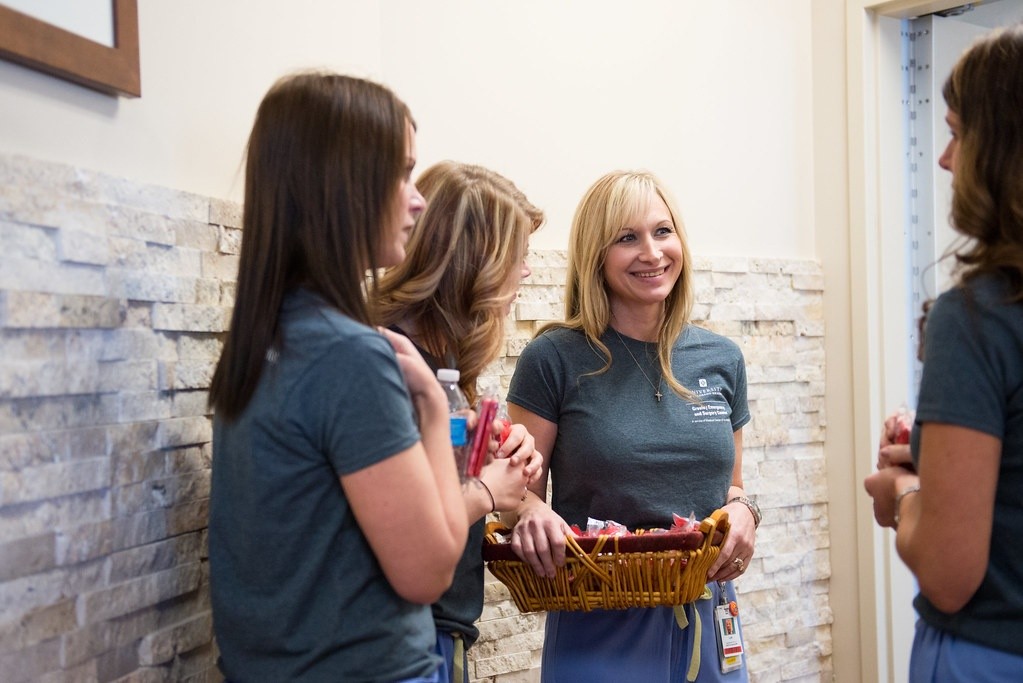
475;476;496;513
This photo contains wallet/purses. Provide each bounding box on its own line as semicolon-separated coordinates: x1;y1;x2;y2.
462;397;498;478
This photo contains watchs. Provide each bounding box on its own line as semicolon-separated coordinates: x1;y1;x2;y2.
726;497;762;531
894;483;919;522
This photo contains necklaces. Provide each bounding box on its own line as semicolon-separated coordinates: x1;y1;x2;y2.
611;323;664;402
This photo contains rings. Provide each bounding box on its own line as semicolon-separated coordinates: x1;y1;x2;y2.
525;486;528;494
733;558;743;568
738;565;744;572
521;495;527;501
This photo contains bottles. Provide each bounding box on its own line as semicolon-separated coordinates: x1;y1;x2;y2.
437;368;470;488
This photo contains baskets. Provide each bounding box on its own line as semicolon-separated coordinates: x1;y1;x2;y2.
483;509;734;616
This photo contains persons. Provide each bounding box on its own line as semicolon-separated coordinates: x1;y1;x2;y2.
864;27;1023;683
499;171;762;683
206;73;546;683
725;618;735;635
